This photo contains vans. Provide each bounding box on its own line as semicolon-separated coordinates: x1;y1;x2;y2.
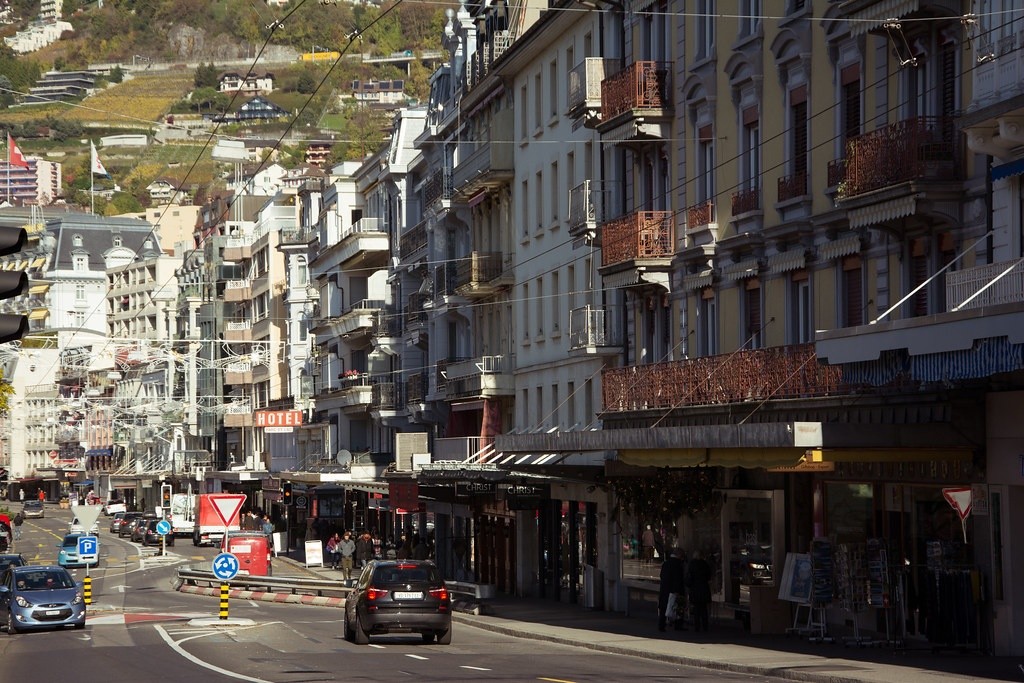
219;530;272;587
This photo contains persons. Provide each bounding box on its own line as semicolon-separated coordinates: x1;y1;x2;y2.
16;574;26;588
688;549;712;631
14;513;23;540
327;531;411;570
140;498;146;511
244;509;274;545
18;489;25;504
37;488;45;502
658;547;689;632
42;574;55;587
641;524;655;563
338;532;356;580
413;537;428;560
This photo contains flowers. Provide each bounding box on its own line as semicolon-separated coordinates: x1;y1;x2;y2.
337;370;357;379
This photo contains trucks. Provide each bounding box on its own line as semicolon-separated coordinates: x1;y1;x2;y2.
193;493;240;548
171;493;197;537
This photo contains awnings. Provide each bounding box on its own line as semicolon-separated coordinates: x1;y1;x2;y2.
21;309;50;319
7;257;46;270
30;285;50;294
86;449;112;456
73;481;94;486
27;236;40;241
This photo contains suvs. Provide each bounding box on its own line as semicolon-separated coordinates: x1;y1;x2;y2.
118;512;143;539
343;559;453;646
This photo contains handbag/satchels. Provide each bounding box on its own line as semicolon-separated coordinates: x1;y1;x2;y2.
327;545;332;552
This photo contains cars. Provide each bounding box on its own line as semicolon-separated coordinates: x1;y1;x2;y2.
67;517;100;539
0;553;27;578
0;532;13;555
21;500;45;520
143;510;157;519
103;499;127;516
131;517;147;543
142;519;175;546
0;565;87;635
108;513;124;533
739;544;773;585
56;533;101;570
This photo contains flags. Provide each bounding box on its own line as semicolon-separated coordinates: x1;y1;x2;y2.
8;136;30;170
92;145;112;180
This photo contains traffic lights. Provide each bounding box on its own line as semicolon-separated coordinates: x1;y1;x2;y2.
283;482;293;506
161;485;172;509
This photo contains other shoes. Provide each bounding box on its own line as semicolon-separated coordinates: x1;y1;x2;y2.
336;566;339;568
332;566;335;570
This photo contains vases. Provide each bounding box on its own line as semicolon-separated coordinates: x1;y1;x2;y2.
343;376;352;379
353;375;357;379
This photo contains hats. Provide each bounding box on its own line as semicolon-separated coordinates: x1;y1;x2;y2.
344;532;350;536
